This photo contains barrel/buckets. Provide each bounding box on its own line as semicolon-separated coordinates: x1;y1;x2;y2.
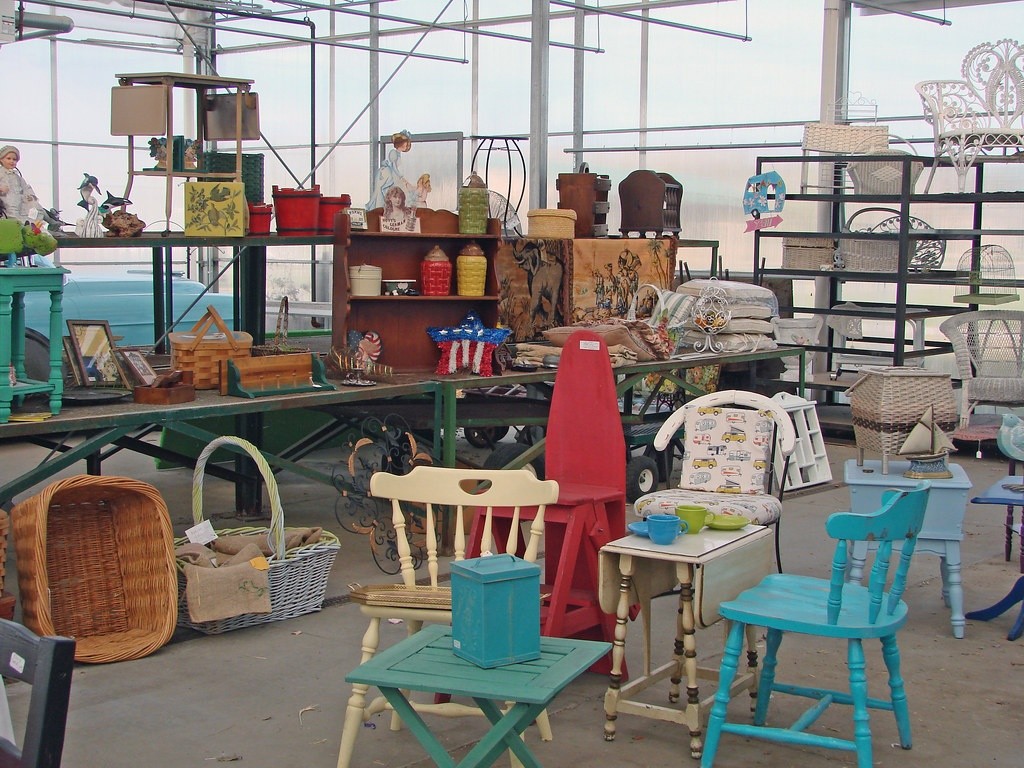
246;201;273;236
319;193;352;235
271;185;320;236
349;259;382;296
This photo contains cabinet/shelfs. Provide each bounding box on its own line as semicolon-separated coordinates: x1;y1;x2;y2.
556;162;612;239
0;232;723;520
769;391;833;491
754;156;1024;456
332;209;502;373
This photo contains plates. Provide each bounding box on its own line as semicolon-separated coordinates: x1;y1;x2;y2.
706;514;751;530
627;521;648;537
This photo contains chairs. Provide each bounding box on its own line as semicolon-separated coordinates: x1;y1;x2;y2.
939;310;1024;428
701;479;932;768
337;467;558;767
633;389;796;574
466;328;642;683
0;617;77;768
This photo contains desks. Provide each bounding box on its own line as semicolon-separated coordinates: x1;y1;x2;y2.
597;525;774;759
0;266;72;421
344;623;613;767
110;73;259;229
0;380;442;556
843;459;972;639
425;346;805;558
964;476;1024;640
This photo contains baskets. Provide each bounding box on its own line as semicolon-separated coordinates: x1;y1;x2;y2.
254;296;309;356
173;434;340;635
847;134;924;195
11;475;178;664
203;152;264;205
840;208;917;273
168;306;254;389
803;121;889;153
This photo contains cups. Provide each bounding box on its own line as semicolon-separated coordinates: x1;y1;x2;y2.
646;514;688;546
675;504;715;534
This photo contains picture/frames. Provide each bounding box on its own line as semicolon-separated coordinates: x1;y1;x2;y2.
61;319;157;390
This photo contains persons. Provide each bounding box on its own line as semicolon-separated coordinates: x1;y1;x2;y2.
0;145;44;224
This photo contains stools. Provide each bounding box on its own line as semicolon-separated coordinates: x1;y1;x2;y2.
471;135;528;239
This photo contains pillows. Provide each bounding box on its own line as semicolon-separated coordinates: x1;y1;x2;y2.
541;277;779;361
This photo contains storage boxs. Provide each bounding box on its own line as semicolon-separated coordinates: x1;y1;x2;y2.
448;552;541;669
405;458;477;533
